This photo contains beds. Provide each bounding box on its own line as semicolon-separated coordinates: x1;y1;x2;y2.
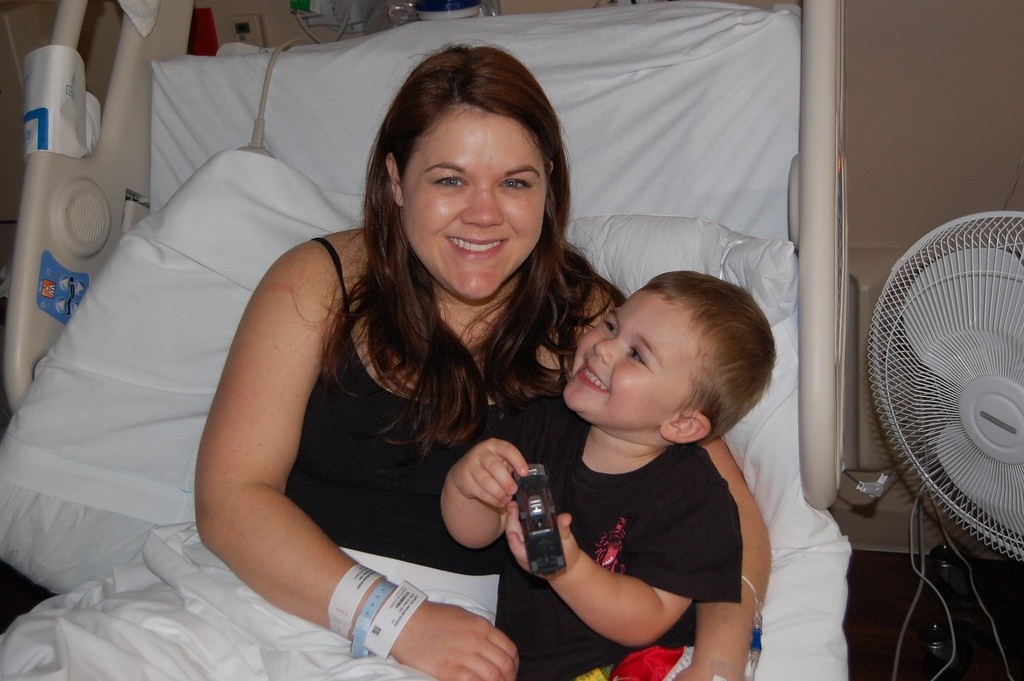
5;0;849;681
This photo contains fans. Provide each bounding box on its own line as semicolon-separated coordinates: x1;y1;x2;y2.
866;210;1023;681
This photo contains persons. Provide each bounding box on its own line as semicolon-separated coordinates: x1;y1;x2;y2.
441;271;776;681
196;45;772;681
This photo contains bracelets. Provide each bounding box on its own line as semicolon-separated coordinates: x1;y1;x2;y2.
327;566;428;660
